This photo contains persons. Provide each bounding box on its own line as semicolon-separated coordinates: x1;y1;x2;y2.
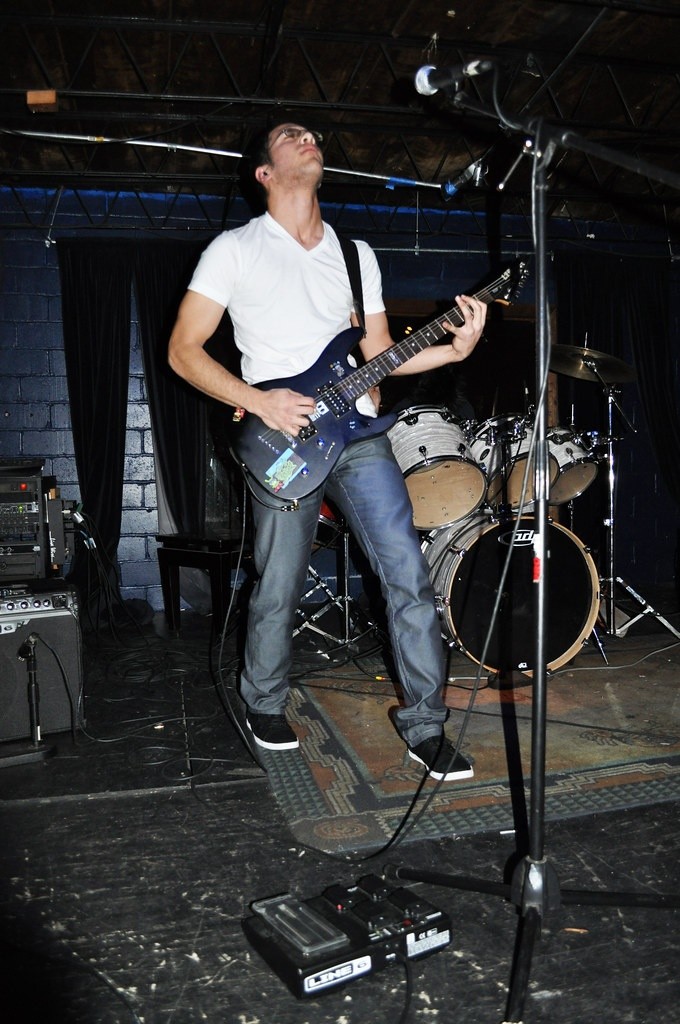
168;121;488;782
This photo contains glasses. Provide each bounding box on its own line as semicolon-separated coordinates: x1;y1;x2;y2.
268;128;324;151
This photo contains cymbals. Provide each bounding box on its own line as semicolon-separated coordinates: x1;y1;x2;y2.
547;344;638;384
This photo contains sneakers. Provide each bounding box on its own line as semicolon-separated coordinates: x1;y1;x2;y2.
407;737;475;781
245;710;300;750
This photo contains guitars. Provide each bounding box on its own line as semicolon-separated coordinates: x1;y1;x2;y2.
227;258;534;501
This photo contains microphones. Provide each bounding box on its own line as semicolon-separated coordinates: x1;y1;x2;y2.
414;60;494;96
19;632;39;658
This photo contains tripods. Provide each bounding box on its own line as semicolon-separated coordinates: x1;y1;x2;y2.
395;86;680;1024
290;520;393;652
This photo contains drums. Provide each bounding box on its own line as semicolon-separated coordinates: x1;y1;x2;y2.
385;404;489;531
468;412;560;509
544;426;599;505
317;497;348;533
419;511;602;678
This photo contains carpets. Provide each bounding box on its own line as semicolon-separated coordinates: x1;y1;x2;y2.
224;633;679;853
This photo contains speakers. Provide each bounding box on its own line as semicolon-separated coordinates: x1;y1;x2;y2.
0;590;86;743
